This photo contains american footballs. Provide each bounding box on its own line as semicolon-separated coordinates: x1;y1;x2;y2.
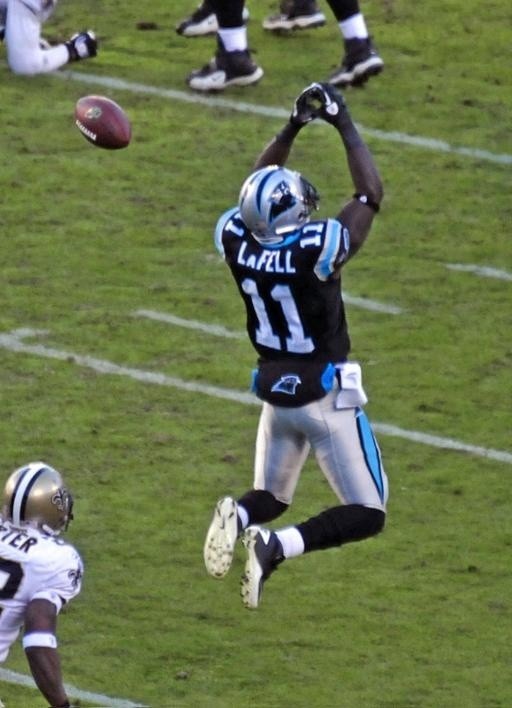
74;95;136;149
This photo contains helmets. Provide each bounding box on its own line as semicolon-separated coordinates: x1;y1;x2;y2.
237;164;322;239
0;460;76;539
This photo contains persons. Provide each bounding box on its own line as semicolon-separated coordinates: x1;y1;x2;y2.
175;0;264;91
203;81;390;610
0;0;99;77
0;462;84;708
265;1;384;90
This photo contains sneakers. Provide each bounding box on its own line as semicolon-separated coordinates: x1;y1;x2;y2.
173;0;250;38
238;523;287;612
201;495;244;582
185;46;266;92
262;0;328;33
322;37;386;90
